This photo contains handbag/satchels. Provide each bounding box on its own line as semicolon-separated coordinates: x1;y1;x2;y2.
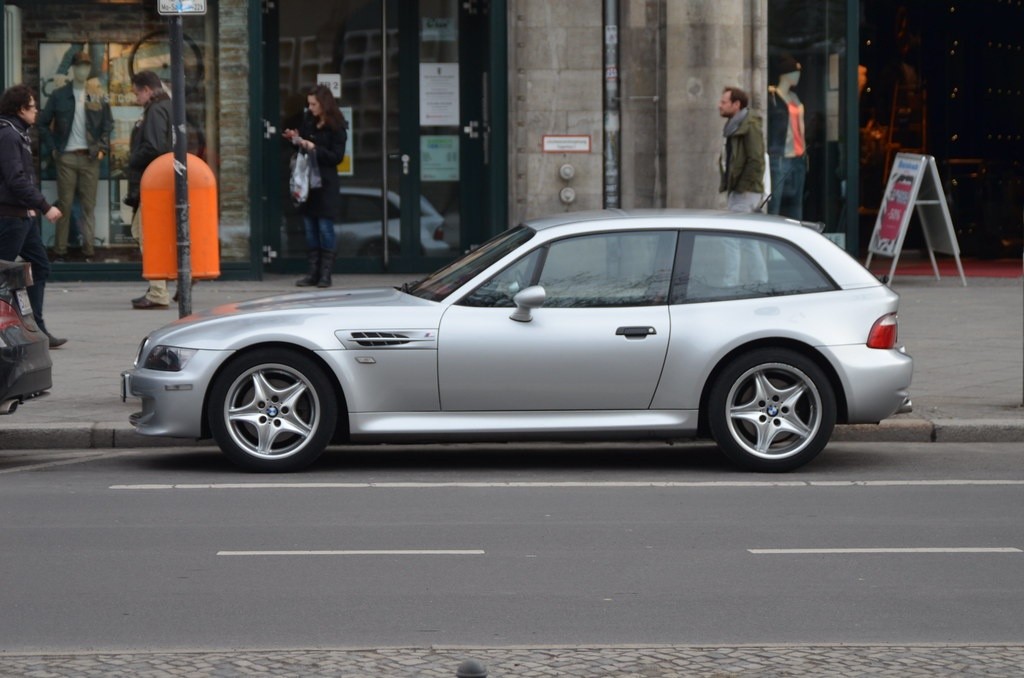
289;138;322;204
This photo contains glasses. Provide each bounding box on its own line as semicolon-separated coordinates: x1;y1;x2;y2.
20;102;39;107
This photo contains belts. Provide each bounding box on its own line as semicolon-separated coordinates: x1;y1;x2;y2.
70;149;89;156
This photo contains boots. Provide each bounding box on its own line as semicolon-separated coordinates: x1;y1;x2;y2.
297;247;337;289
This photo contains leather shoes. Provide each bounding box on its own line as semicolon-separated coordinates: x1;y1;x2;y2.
131;294;167;311
38;332;67;347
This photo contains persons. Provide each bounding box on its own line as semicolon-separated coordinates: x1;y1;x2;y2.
122;70;199;308
715;86;765;212
768;52;809;221
0;84;67;348
40;53;114;263
283;87;347;287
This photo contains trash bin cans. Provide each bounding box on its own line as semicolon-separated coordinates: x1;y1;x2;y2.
138;152;223;280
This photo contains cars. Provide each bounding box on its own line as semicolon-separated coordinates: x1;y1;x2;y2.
119;206;917;476
0;261;54;414
213;185;450;271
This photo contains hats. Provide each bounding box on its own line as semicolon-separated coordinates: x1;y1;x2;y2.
71;52;93;64
